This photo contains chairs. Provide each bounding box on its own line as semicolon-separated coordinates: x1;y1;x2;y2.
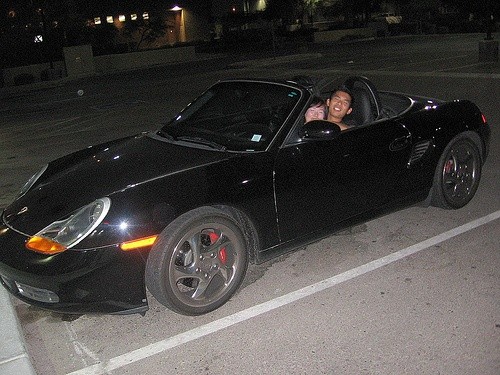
341;86;372;126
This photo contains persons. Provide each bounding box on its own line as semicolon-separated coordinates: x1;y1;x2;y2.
325;88;354;130
303;98;328;125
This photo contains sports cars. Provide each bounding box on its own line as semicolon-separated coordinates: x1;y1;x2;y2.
0;76;490;316
